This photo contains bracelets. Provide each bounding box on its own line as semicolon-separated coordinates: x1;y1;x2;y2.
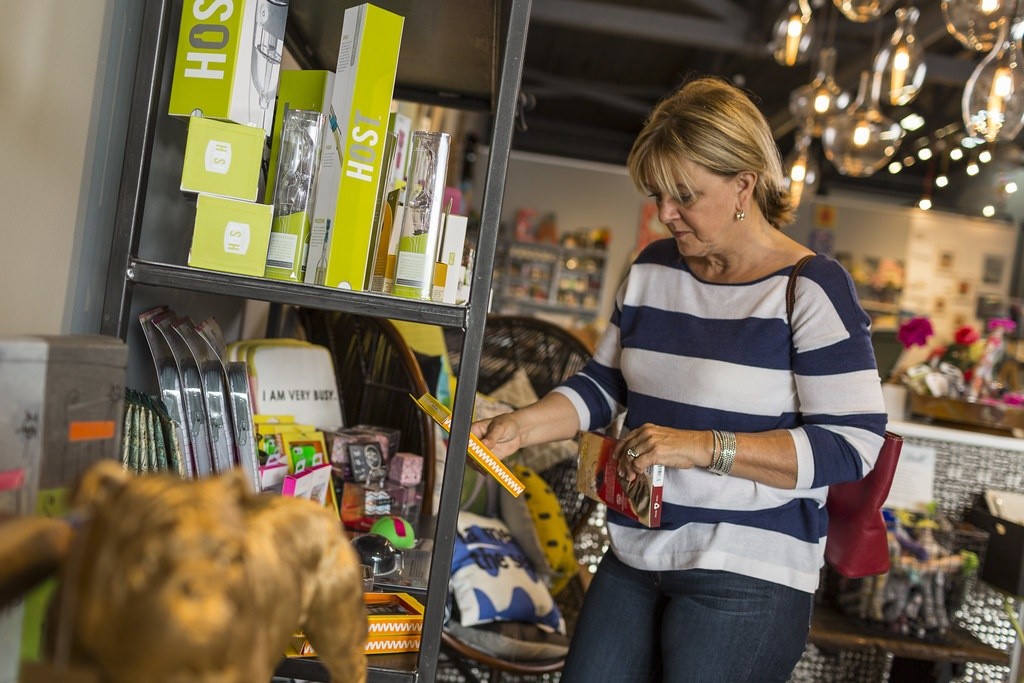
706;430;737;476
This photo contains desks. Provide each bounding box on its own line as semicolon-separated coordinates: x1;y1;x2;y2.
814;610;1024;683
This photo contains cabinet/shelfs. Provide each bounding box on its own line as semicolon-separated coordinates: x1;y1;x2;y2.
111;0;534;683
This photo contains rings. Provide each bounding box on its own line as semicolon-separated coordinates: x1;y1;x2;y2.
628;448;640;458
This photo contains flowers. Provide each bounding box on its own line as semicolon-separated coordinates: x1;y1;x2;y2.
897;312;1016;383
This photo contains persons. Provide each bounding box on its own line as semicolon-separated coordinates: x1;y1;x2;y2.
466;79;887;682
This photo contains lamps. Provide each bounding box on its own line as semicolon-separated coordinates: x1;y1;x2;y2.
739;1;1024;208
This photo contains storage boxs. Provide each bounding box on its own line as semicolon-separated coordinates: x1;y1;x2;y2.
290;593;426;658
165;0;479;303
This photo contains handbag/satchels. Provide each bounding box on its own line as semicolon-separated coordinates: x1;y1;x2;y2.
786;254;904;578
